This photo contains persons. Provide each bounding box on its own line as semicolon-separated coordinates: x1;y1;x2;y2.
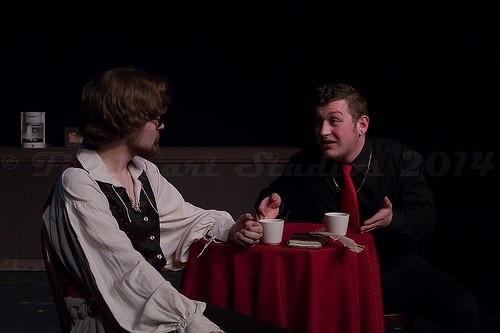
255;83;480;333
42;67;290;333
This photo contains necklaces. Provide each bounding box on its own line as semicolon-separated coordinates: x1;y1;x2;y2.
333;148;372;194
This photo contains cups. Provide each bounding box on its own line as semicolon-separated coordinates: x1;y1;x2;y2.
324;212;350;236
258;219;284;245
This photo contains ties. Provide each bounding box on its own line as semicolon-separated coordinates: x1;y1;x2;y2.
340;164;360;230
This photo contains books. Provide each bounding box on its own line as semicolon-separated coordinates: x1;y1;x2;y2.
287;232;326;250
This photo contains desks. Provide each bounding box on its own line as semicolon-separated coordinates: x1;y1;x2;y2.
181;223;385;333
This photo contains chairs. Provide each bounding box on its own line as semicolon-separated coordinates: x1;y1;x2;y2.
383;299;419;333
40;226;73;333
